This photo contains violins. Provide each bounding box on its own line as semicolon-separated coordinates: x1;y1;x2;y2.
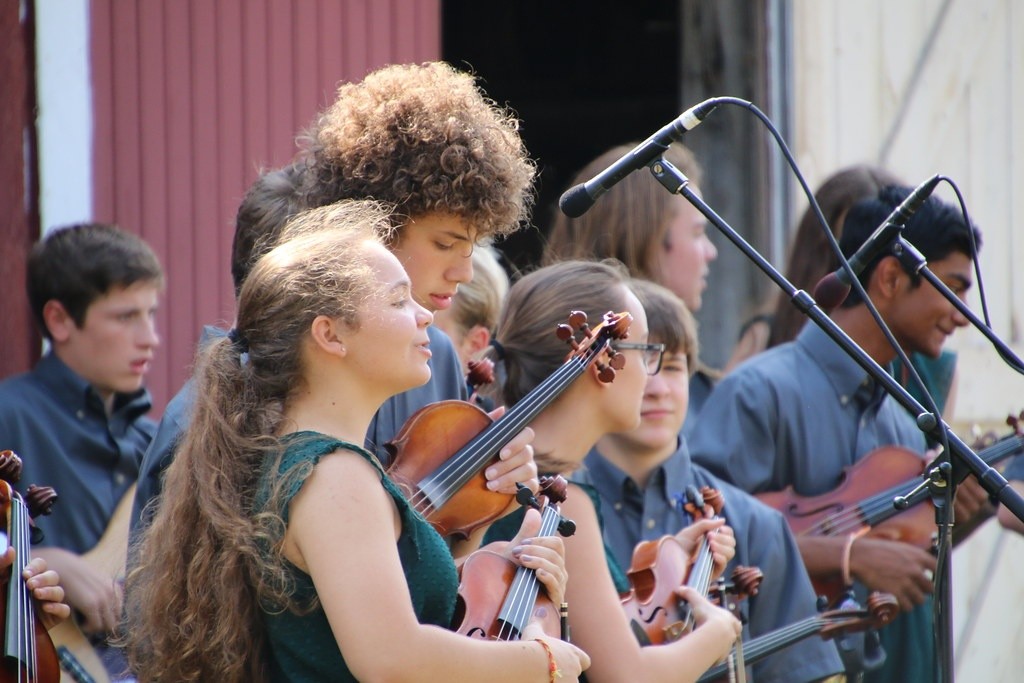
750;409;1024;606
0;451;60;682
618;485;727;648
695;591;900;683
391;310;635;543
452;475;576;640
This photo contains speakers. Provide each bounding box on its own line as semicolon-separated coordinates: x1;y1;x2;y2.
439;1;686;286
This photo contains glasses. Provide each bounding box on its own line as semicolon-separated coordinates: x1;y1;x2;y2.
610;342;665;375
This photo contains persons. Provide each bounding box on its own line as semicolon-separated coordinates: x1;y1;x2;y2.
0;61;1024;683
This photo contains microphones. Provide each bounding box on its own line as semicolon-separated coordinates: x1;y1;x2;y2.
557;97;720;219
810;174;943;311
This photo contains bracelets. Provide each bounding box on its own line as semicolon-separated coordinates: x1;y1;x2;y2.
841;525;871;586
530;638;562;683
